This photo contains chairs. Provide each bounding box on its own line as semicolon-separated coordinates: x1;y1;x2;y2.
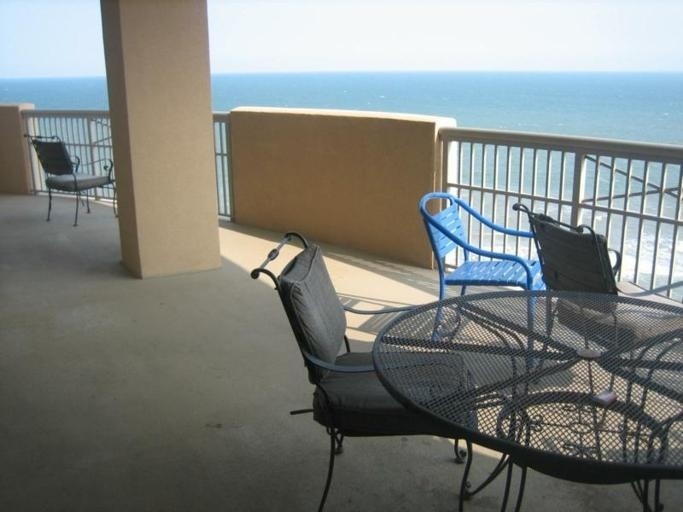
512;204;682;428
419;192;547;370
250;231;479;511
23;133;119;227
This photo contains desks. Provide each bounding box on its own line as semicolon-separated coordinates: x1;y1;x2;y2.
372;292;682;511
